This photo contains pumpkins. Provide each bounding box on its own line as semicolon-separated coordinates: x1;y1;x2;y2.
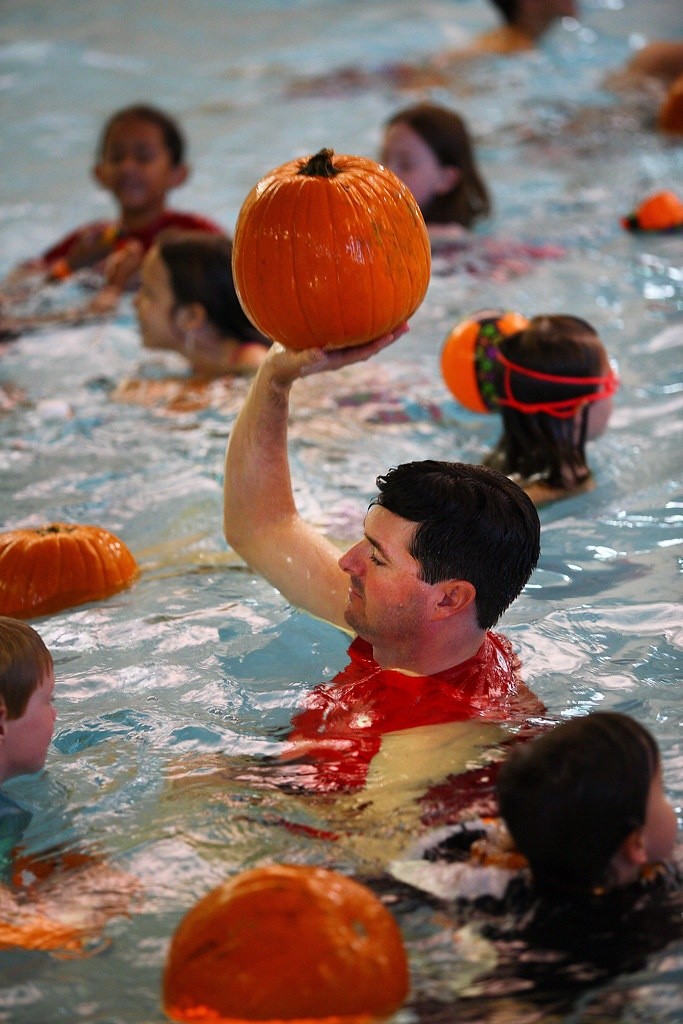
0;523;140;622
163;862;410;1024
232;146;432;353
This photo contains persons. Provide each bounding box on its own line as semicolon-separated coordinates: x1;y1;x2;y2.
380;105;485;233
39;104;220;290
224;323;545;730
486;313;614;498
499;710;678;895
0;616;54;781
134;233;268;373
479;0;574;53
